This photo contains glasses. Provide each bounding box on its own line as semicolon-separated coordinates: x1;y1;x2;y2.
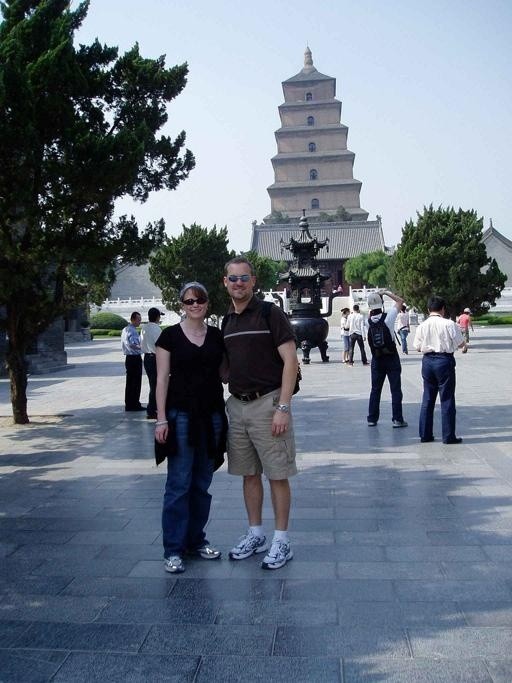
228;274;249;282
183;296;207;305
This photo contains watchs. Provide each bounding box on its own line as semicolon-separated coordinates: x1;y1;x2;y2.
276;404;289;412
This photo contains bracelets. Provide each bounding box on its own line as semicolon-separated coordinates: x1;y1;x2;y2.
156;421;169;426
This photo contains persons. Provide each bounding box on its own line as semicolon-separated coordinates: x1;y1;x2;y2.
367;291;409;428
397;305;411;355
413;296;463;445
143;308;164;417
350;305;369;365
339;307;349;363
155;283;223;574
458;307;475;353
122;312;148;413
221;256;298;570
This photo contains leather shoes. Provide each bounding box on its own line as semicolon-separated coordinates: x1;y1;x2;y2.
126;405;146;411
421;436;434;442
443;437;462;444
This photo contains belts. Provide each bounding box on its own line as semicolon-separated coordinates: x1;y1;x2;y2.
231;385;280;402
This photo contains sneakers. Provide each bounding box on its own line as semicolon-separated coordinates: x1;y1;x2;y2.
164;556;186;573
229;529;269;559
184;544;221;560
368;420;376;426
260;537;292;569
392;420;408;427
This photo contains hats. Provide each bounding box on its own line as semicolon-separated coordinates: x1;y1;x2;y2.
464;308;472;315
367;293;383;310
148;308;165;315
178;278;208;299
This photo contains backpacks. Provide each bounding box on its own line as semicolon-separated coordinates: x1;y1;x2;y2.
369;312;393;354
